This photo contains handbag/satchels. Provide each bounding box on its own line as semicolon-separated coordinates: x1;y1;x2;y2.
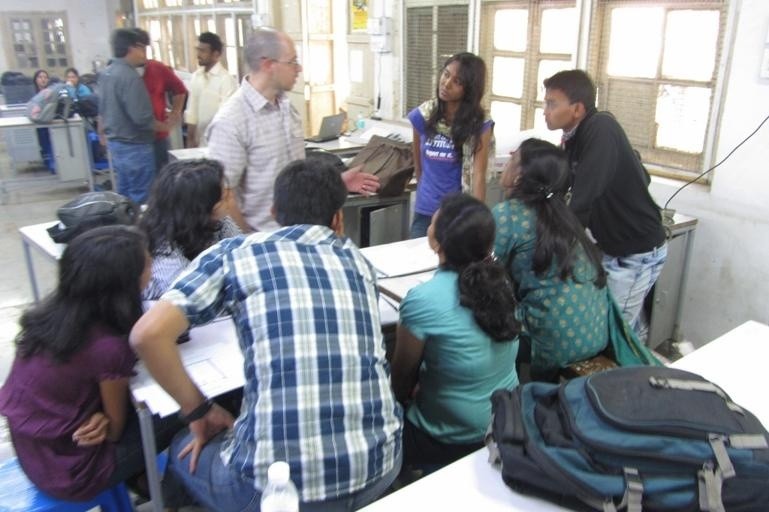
348;134;414;196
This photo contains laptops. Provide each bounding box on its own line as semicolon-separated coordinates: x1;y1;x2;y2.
305;112;346;142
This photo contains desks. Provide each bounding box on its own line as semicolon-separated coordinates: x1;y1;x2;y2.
1;101;769;512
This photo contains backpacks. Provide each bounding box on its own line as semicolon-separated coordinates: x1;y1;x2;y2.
47;191;139;243
27;83;99;124
486;366;769;512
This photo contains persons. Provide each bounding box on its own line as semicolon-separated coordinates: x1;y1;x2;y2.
389;192;521;465
492;136;668;383
133;158;244;301
542;67;669;329
205;26;382;236
31;25;239;202
1;224;178;503
407;51;495;239
126;159;405;512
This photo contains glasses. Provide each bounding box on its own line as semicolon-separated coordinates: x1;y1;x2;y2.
262;57;300;66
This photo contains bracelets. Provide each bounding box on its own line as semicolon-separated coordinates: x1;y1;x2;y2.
185;399;213;424
